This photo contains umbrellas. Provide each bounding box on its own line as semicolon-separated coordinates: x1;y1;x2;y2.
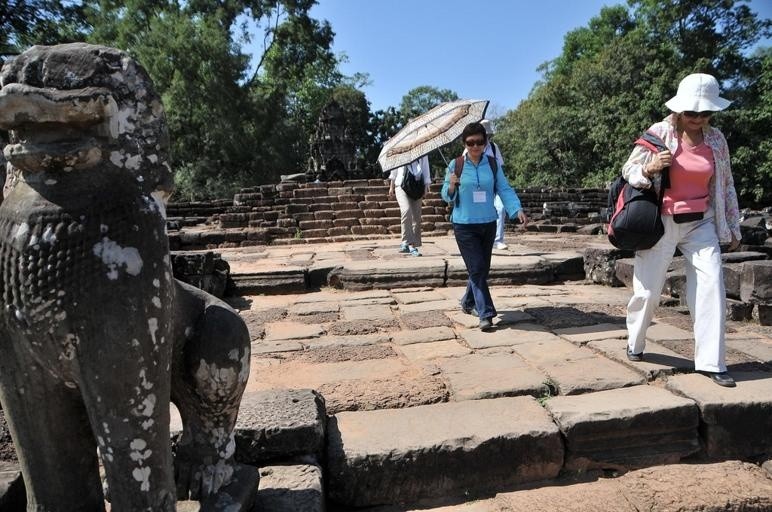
376;99;490;185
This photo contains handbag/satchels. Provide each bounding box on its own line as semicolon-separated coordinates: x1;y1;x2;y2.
400;168;426;201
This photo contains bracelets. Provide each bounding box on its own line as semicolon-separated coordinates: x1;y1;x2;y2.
643;162;650;176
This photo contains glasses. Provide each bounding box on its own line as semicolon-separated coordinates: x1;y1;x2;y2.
465;139;486;147
683;111;716;119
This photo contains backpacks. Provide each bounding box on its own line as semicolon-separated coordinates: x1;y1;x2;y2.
604;174;665;251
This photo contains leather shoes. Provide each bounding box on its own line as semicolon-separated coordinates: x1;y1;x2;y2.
627;345;643;361
706;372;736;387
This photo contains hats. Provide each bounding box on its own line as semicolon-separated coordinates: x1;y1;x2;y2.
665;72;734;115
479;120;494;138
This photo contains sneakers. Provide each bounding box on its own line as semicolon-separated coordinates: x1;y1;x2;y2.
478;318;493;331
494;240;509;252
410;247;421;257
399;242;410;253
462;305;482;318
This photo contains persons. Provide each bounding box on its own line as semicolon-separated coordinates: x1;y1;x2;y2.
463;119;508;250
441;123;529;331
623;73;742;387
388;154;431;257
